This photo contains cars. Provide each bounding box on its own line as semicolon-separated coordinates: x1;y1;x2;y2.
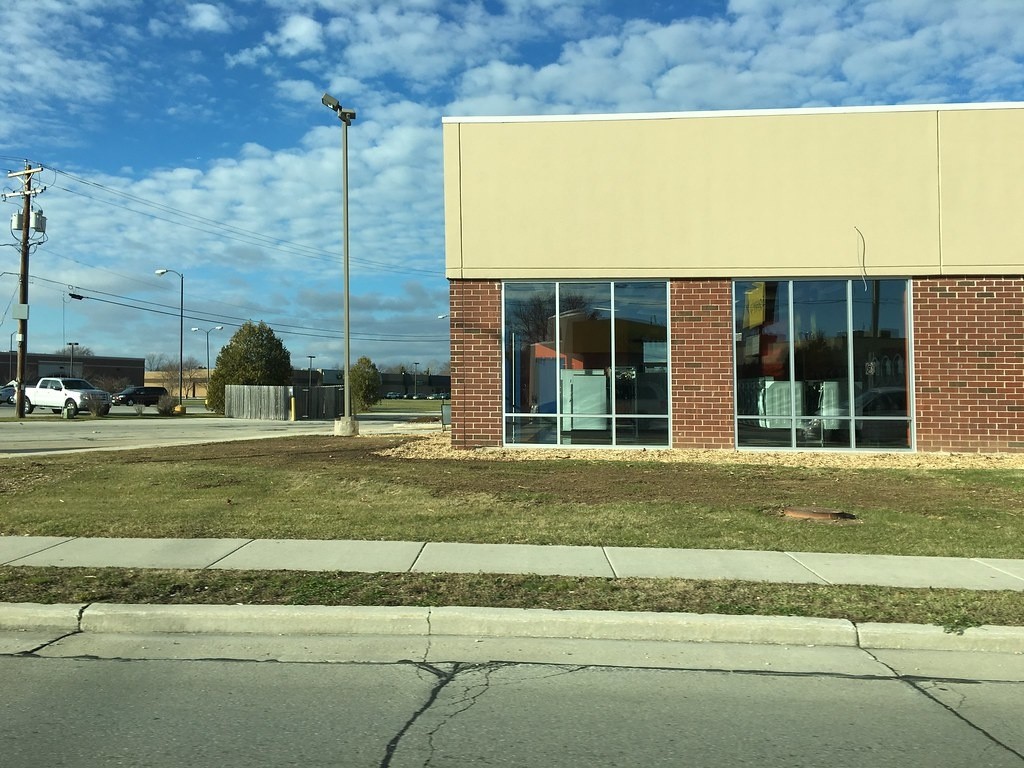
387;392;450;400
809;385;905;441
111;386;168;407
0;385;15;404
532;382;667;433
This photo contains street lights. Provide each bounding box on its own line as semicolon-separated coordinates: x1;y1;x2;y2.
191;326;223;383
155;269;183;405
321;94;354;420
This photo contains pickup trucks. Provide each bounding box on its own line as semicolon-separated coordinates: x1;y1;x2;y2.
25;378;112;416
